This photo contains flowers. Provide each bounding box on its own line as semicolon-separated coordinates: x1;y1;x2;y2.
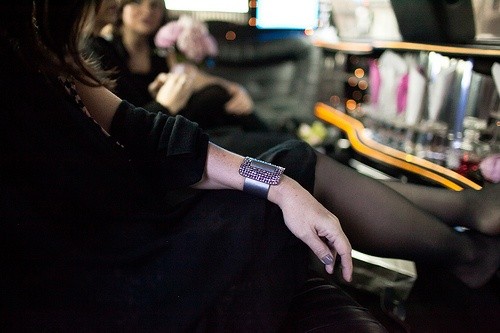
157;14;221;74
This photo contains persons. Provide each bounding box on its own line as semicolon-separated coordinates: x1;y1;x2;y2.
94;0;176;86
122;70;264;135
1;0;353;333
209;128;499;286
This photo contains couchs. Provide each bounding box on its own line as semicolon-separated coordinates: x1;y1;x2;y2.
204;34;325;130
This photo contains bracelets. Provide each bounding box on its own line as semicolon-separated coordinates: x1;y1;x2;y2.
239;155;286;198
